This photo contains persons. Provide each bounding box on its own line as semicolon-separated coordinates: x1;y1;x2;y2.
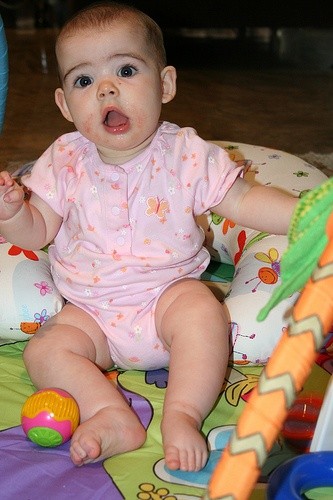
1;5;316;472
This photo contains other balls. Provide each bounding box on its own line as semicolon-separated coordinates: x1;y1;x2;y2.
21;388;79;447
